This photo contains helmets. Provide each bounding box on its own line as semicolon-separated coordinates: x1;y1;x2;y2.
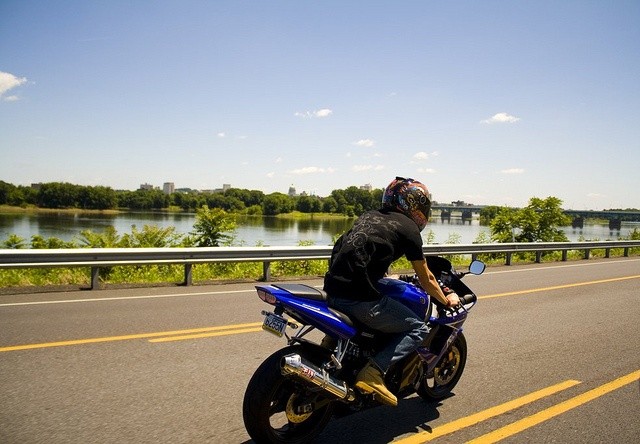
382;178;431;231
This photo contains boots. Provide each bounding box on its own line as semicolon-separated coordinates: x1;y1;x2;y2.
356;360;398;407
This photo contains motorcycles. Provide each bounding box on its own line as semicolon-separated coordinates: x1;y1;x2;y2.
243;255;486;444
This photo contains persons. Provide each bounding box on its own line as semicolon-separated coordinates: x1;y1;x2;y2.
323;176;463;407
